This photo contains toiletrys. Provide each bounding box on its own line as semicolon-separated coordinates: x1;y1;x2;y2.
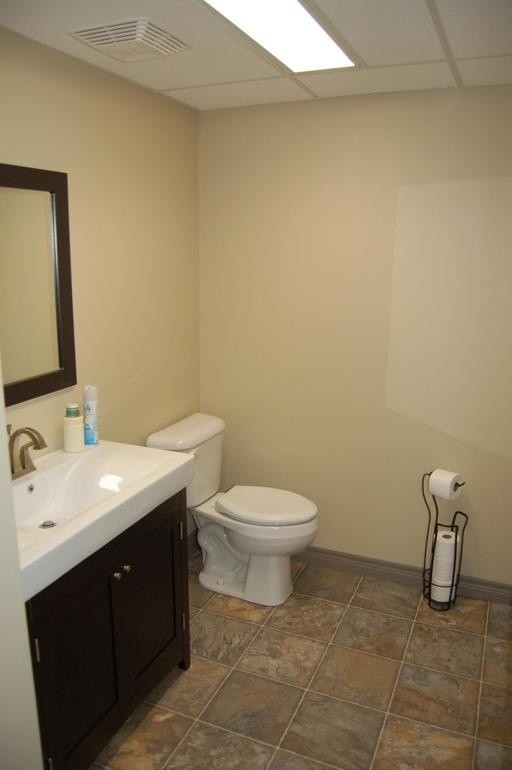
62;403;86;453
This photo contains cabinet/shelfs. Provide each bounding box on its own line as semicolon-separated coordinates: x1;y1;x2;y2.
24;489;192;767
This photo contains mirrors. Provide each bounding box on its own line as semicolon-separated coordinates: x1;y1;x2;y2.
0;162;79;407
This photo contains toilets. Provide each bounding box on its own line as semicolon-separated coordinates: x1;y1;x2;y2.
147;412;322;608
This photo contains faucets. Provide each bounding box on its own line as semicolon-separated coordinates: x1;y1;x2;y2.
8;423;48;479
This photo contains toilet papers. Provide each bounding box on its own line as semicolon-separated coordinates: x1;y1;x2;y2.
428;468;463;504
432;530;462;605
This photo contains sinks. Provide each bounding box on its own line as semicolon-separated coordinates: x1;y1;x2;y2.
14;440;192;604
